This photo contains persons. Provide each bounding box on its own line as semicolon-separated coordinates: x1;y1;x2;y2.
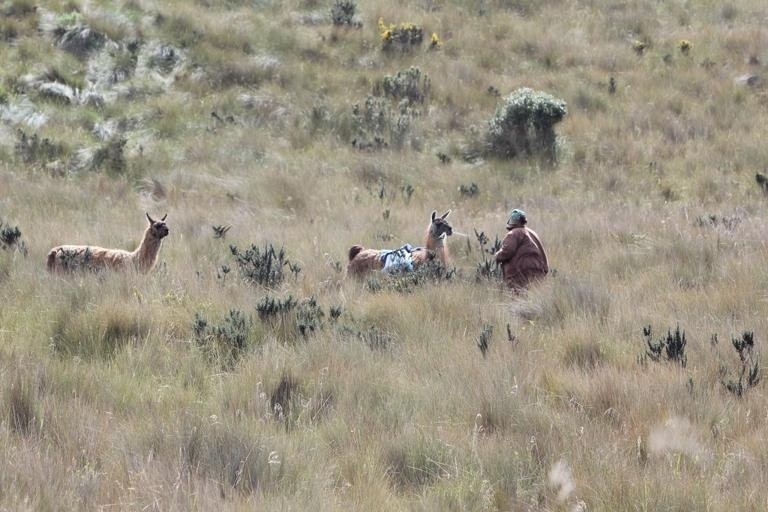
497;208;549;288
